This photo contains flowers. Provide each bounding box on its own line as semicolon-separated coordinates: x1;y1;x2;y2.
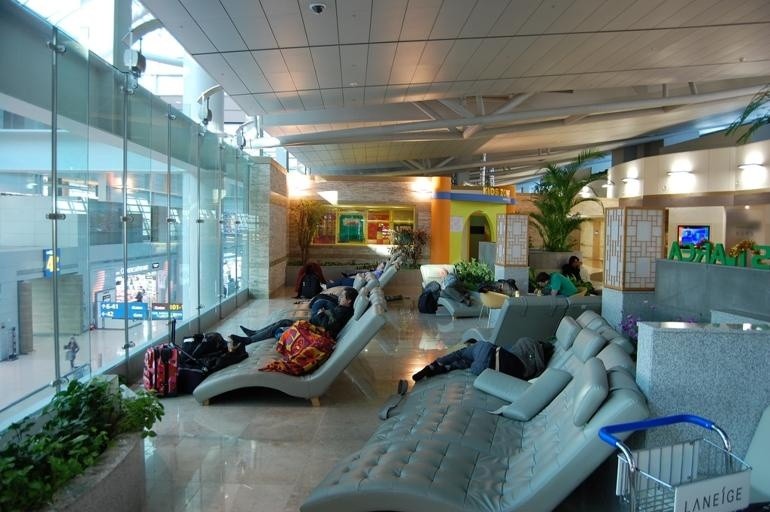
614;301;701;344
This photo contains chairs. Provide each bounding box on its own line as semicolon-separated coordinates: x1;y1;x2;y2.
419;260;603;353
189;253;404;407
297;307;652;509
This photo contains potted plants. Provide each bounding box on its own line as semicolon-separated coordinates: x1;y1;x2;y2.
526;143;607;270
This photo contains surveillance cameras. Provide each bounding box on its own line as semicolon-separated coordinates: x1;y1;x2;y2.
309;3;326;15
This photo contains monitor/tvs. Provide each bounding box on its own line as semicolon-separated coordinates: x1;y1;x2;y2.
677;225;711;250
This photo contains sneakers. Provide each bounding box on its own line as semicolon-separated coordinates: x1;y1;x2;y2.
410;368;431;381
230;335;251;345
465;298;471;306
240;326;257;336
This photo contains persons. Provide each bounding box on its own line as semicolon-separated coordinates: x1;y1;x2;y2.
562;256;600;295
439;268;471;306
231;262;386;345
412;336;552;381
64;338;79;368
536;272;578;296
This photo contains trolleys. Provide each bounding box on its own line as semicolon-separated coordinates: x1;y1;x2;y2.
598;414;752;512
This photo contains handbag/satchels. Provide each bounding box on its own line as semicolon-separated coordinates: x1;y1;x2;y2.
418;281;440;314
180;332;226;367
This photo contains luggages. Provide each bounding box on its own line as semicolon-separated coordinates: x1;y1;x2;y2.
300;262;323;297
143;319;177;398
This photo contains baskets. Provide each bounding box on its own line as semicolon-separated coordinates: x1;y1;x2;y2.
615;438;752;512
